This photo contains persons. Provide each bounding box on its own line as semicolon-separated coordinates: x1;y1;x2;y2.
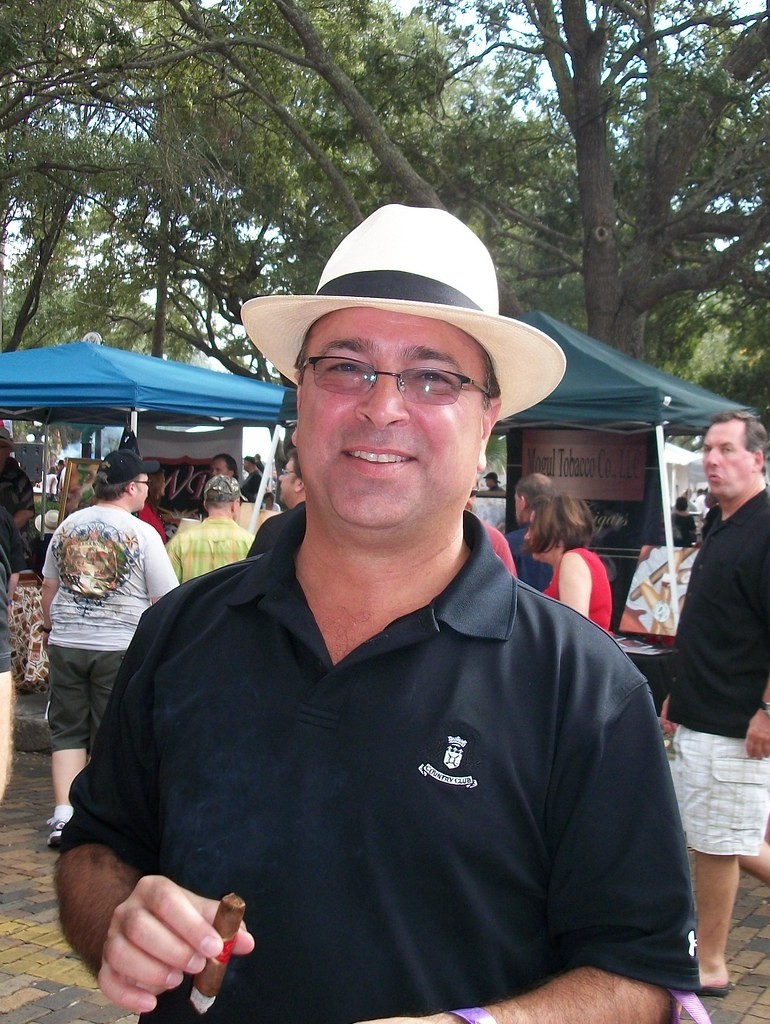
682;488;721;540
246;446;307;558
660;413;770;999
131;460;168;545
54;203;700;1023
241;453;280;512
670;497;697;547
38;449;180;843
165;475;256;586
465;473;612;631
483;472;505;492
0;426;34;804
199;454;254;520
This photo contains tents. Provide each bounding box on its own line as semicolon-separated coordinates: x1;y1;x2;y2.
497;310;759;630
0;339;300;440
665;441;701;506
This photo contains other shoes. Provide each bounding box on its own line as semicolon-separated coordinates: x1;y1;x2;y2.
45;818;67;845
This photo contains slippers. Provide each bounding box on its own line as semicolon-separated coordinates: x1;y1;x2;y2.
693;984;729;997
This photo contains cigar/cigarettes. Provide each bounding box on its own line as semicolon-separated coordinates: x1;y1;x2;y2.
191;892;245;1013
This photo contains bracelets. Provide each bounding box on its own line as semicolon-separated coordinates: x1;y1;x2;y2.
760;699;770;715
448;1005;497;1024
41;625;52;634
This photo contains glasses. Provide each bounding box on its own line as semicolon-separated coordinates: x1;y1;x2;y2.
282;468;298;477
299;355;493;406
124;480;152;491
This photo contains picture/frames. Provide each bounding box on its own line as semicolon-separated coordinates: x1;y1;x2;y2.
56;457;105;527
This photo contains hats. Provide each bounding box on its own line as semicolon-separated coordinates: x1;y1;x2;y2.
240;203;566;422
244;456;256;463
0;427;16;451
94;449;161;484
204;474;249;502
